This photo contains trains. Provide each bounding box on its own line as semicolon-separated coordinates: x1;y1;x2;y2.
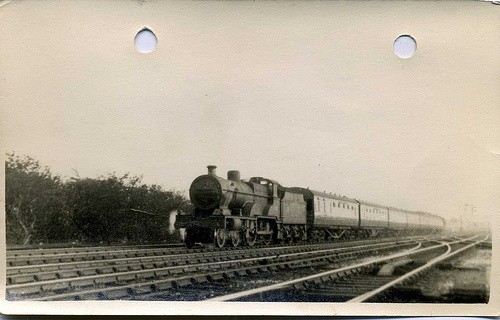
173;165;445;250
